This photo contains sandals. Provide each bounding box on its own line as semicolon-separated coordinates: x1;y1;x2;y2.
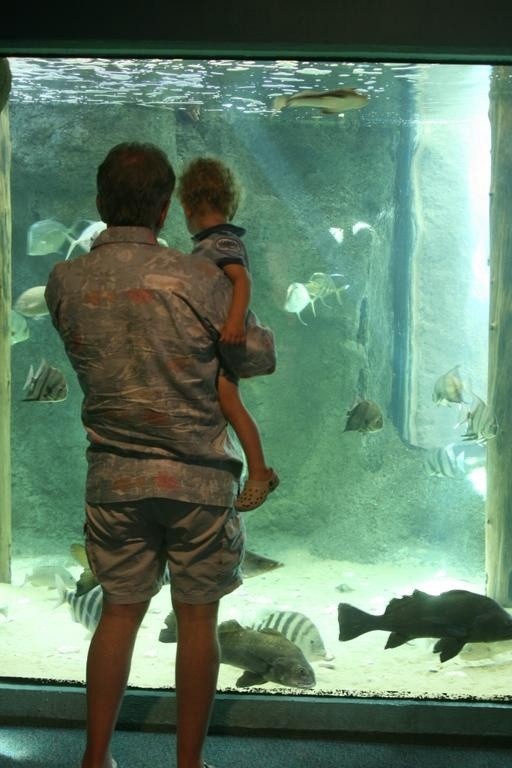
234;467;280;512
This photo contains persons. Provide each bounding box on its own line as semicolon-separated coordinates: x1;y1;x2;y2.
46;145;279;766
178;159;278;512
